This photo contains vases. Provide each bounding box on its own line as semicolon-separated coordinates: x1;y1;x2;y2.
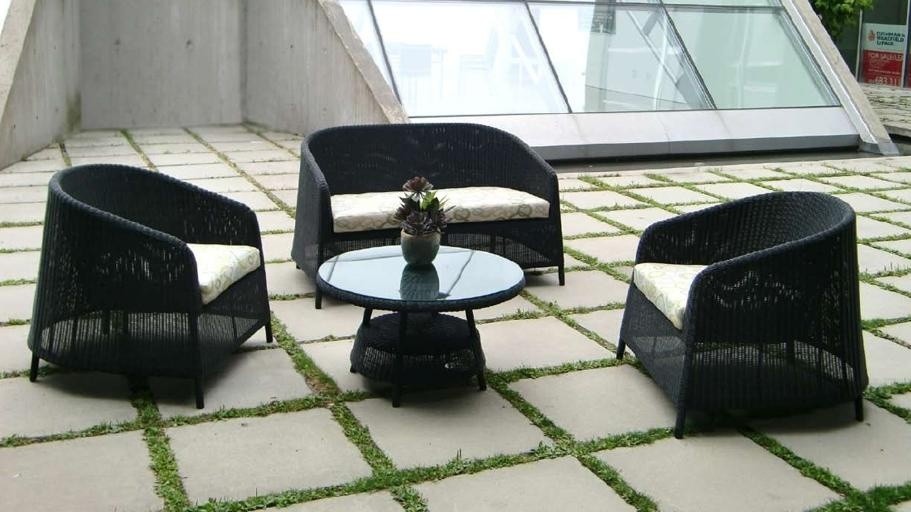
401;229;440;268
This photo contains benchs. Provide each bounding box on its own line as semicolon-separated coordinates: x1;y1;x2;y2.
292;123;565;310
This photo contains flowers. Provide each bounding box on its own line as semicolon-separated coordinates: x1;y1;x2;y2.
392;176;456;236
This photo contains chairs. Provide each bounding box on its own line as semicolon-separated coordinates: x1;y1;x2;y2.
617;192;869;439
27;164;273;409
